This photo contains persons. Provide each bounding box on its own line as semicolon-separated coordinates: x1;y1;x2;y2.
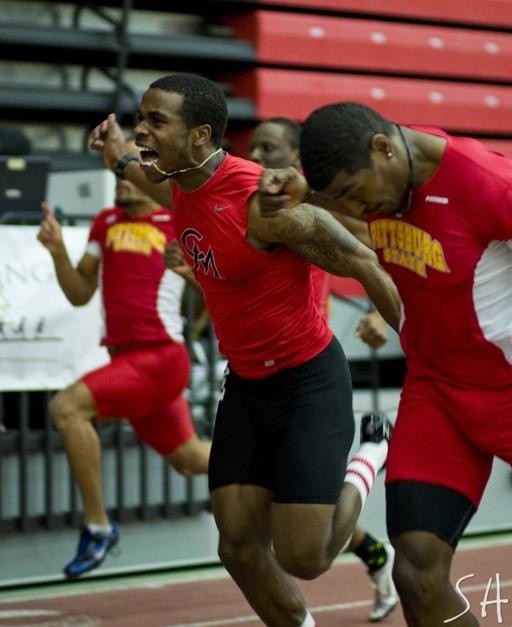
39;134;215;575
242;115;401;622
87;73;402;627
257;102;512;625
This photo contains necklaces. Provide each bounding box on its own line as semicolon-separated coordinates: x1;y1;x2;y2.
153;147;223;180
396;126;414;218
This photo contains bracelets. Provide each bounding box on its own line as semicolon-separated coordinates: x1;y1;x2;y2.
115;155;137;178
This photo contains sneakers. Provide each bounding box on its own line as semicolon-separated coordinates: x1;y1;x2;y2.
63;522;119;578
360;411;393;472
367;543;400;621
